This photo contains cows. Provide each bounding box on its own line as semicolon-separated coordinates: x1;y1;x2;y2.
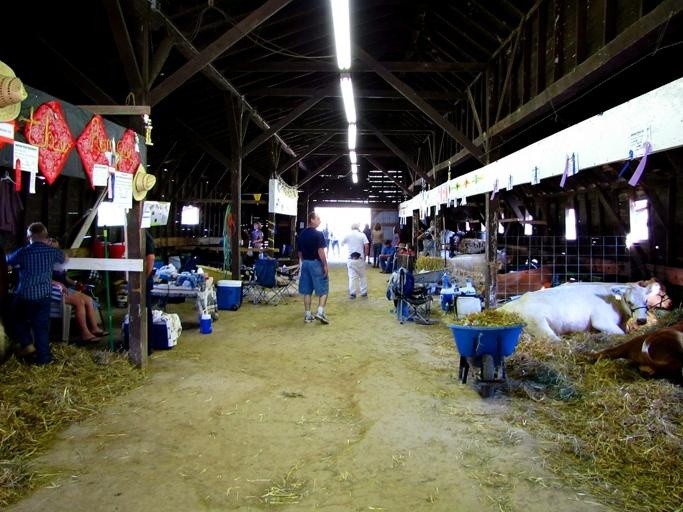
494;268;554;297
554;276;673;307
493;282;651;343
581;320;683;385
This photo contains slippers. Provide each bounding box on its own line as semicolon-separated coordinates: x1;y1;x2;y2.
84;336;102;344
92;330;110;336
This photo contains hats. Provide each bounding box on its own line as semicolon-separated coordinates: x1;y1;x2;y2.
1;63;28;124
132;164;157;201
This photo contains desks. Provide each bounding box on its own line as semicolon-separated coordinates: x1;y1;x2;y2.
151;289;220;327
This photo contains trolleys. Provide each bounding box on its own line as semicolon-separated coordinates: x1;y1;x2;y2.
446;323;522;397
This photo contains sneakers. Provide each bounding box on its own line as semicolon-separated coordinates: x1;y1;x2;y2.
315;310;329;324
304;314;315;323
19;343;36;354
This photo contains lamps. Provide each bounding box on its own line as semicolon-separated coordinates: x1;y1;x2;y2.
330;0;359;184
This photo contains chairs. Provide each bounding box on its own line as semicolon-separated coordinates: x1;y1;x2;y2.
397;269;434;326
49;292;90;343
242;259;300;306
380;253;398;272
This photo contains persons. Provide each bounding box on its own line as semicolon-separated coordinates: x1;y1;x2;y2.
361;220;466;274
342;223;369;299
6;223;68;366
252;221;263;246
295;213;329;325
146;232;156;356
47;238;109;344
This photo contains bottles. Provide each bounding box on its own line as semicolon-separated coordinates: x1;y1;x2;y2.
442;273;450;289
202;313;212;332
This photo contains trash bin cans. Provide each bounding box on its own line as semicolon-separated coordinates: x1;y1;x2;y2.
255;258;277;287
